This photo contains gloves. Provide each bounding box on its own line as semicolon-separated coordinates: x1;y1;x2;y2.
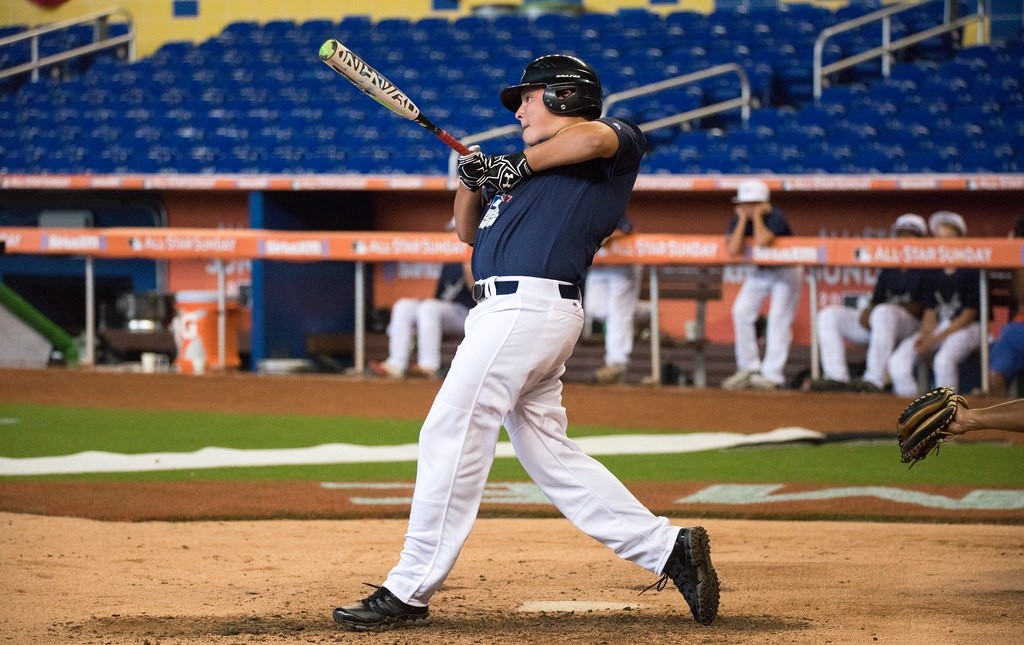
457;145;488;191
483;152;533;198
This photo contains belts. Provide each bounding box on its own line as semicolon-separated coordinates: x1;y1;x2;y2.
471;282;579;301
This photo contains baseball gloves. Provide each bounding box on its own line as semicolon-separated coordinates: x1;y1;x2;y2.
893;384;971;472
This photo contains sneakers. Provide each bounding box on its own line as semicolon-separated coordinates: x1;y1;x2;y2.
637;526;720;625
333;583;431;631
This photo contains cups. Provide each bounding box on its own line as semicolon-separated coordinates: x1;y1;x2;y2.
155;354;170;373
141;352;156;372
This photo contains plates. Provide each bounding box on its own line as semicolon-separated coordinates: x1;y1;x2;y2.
258;358;307;373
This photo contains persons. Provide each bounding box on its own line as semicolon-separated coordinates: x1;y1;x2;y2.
331;53;721;632
887;211;1024;400
716;178;801;389
366;215;478;387
579;213;651;387
806;212;930;394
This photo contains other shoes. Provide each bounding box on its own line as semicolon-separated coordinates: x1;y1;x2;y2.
749;374;778;392
408;364;435;378
852;378;879;392
368;362;393;378
812;380;856;390
595;367;624;385
720;371;759;391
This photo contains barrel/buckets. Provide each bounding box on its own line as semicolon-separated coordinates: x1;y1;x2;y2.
173;289;241;371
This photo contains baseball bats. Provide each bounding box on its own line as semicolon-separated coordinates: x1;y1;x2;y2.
316;37;470;156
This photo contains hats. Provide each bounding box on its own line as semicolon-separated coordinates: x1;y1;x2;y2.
736;180;770;202
893;213;928;235
928;211;968;236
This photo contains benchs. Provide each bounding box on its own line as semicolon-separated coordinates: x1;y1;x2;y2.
0;0;1024;173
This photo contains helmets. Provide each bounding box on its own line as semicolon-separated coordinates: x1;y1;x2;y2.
500;54;602;120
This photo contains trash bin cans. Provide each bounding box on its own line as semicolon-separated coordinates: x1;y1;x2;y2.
174;291;242;372
